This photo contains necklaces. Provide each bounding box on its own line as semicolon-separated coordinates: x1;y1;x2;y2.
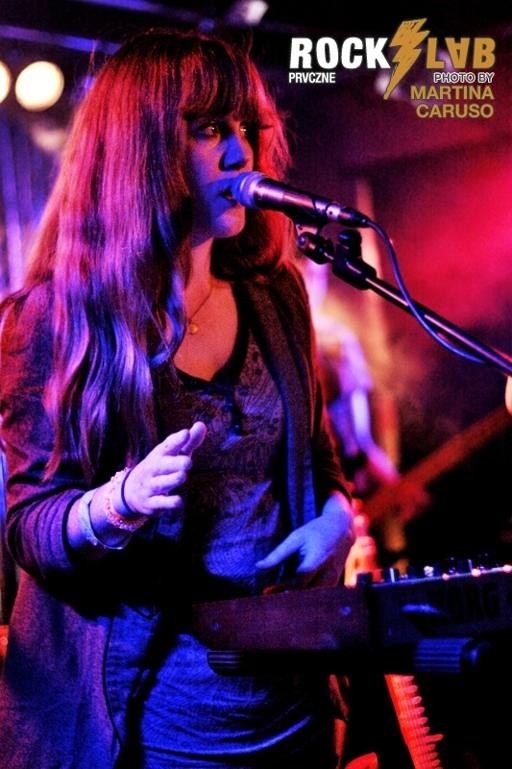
185;274;214;335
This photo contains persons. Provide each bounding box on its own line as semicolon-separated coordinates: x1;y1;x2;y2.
0;29;358;769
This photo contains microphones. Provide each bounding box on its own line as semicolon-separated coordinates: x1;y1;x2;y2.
232;169;373;229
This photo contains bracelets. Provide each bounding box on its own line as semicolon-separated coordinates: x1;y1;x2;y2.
79;467;149;554
